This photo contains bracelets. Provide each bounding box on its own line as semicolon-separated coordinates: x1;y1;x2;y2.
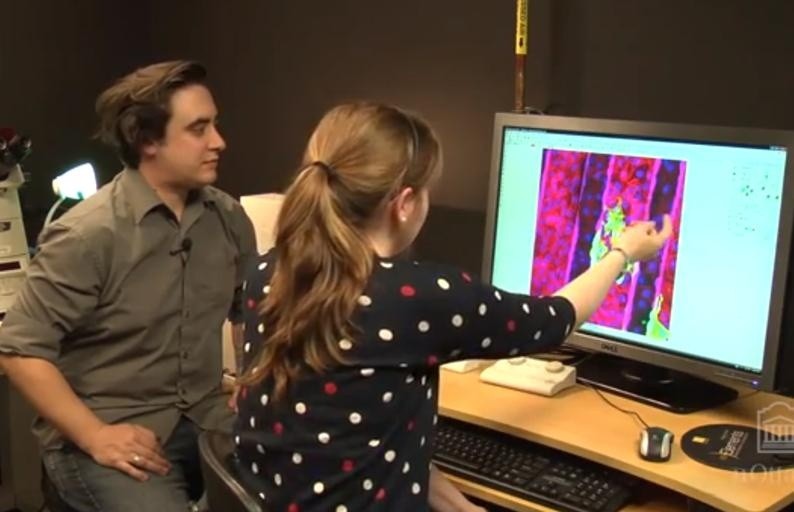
611;248;630;266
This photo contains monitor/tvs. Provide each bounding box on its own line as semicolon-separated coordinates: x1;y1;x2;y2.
480;111;792;413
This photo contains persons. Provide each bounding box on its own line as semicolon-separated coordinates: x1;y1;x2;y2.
235;101;673;512
0;60;259;512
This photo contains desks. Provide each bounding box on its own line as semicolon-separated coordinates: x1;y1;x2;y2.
436;355;793;512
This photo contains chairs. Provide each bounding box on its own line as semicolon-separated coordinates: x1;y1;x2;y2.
192;429;267;511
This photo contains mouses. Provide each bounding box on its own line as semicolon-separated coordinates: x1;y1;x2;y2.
640;426;674;462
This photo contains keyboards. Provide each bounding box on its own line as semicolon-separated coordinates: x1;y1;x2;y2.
429;415;636;511
